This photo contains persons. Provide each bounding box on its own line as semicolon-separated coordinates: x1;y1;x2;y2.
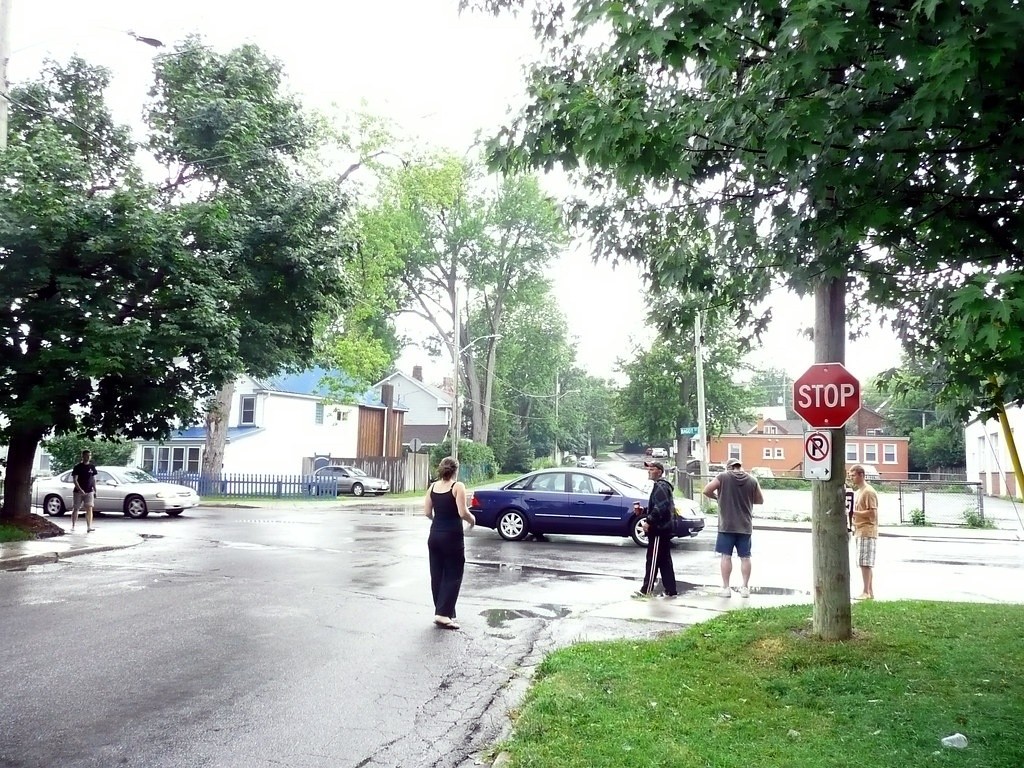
663;460;671;478
702;457;765;597
848;465;880;600
70;449;98;533
633;460;678;600
422;455;475;630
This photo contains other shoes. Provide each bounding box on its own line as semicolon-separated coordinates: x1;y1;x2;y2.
631;591;652;601
717;587;731;597
740;587;749;597
432;615;460;629
657;592;678;600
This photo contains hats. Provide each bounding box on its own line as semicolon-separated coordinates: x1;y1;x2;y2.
644;461;665;472
726;458;742;467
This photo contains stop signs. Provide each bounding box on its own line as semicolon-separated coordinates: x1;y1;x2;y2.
793;361;860;431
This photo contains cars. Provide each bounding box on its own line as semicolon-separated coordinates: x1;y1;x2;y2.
645;447;653;454
576;455;595;468
32;465;200;517
653;447;668;458
302;465;390;498
469;466;706;548
